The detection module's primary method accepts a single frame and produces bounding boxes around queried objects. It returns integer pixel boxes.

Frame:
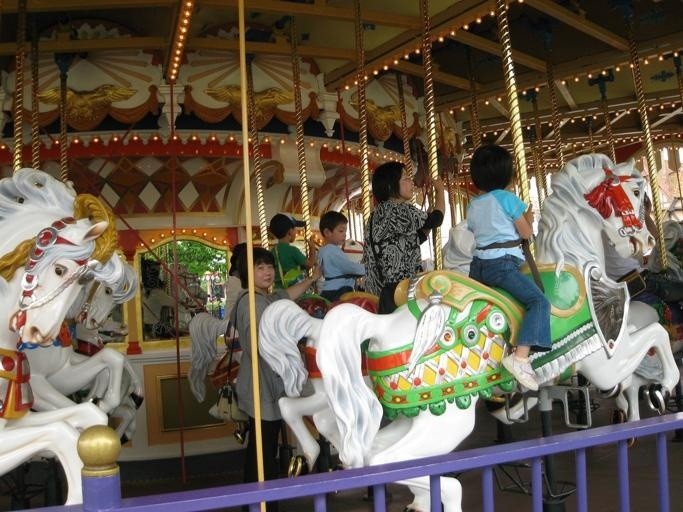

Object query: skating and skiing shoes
[503,352,539,392]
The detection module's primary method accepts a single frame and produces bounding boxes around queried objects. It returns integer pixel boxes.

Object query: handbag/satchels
[377,282,400,313]
[216,383,250,422]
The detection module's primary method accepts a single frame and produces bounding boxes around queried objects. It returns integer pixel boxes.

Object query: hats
[270,213,305,232]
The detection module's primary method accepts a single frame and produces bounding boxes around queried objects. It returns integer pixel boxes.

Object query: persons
[361,160,445,295]
[465,144,552,393]
[270,213,316,288]
[225,243,246,327]
[231,247,321,511]
[141,259,180,339]
[318,211,365,301]
